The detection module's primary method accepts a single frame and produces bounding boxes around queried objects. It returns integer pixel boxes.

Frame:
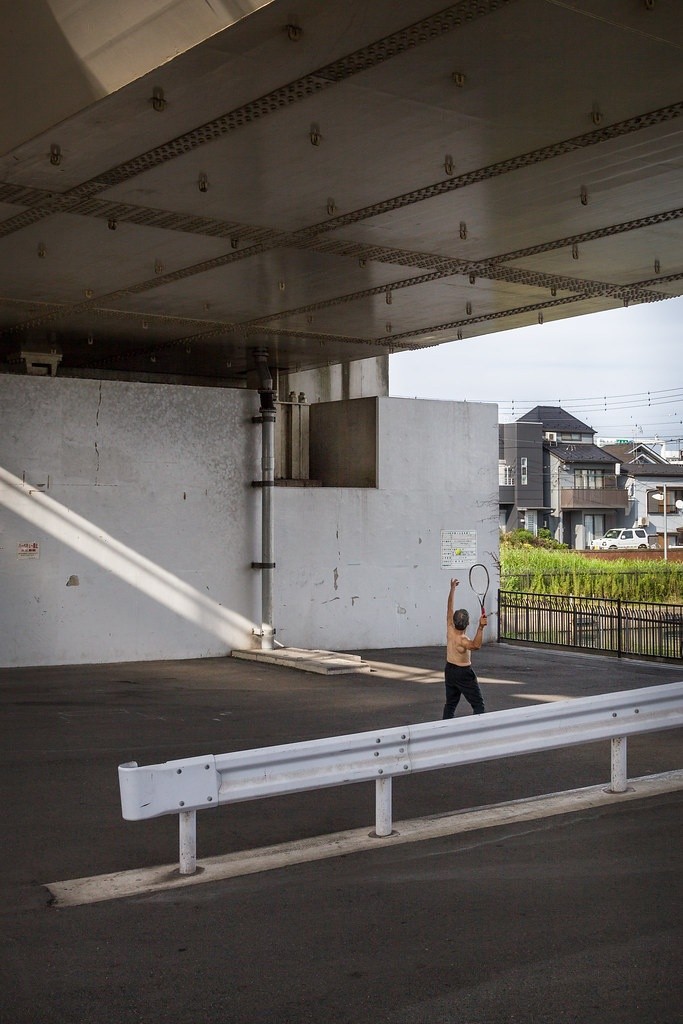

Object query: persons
[442,578,488,720]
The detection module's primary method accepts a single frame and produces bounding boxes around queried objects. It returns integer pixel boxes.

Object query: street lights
[652,492,667,559]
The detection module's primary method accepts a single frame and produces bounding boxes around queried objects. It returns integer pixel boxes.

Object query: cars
[591,528,650,550]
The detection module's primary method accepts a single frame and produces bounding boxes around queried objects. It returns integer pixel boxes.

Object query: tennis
[455,549,462,555]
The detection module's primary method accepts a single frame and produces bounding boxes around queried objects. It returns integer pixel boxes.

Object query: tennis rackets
[469,563,490,626]
